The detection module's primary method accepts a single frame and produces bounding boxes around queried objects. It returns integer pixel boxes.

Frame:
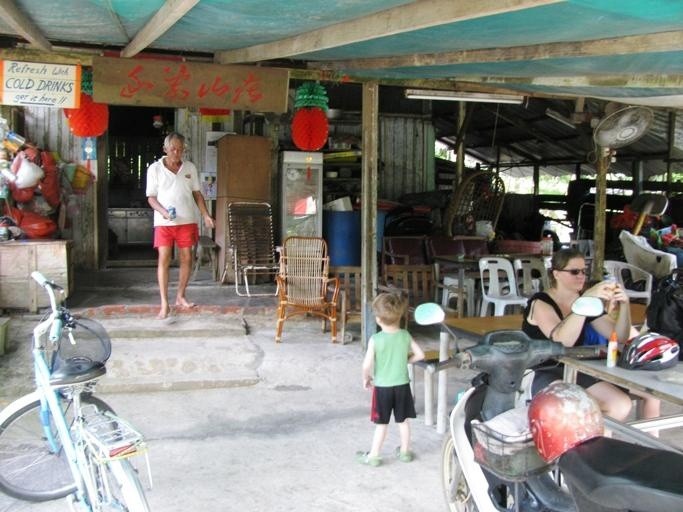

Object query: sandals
[394,445,411,463]
[354,450,380,466]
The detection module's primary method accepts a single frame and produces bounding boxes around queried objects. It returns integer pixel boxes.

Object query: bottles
[0,117,26,201]
[606,332,618,368]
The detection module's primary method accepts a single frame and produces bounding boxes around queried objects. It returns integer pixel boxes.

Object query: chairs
[619,229,677,282]
[274,237,341,340]
[222,200,279,297]
[514,257,548,297]
[603,260,651,304]
[479,257,528,316]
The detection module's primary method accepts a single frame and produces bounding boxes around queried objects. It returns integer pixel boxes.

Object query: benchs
[328,264,439,345]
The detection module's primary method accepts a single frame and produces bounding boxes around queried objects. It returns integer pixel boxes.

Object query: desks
[437,302,659,435]
[555,350,683,487]
[431,251,551,318]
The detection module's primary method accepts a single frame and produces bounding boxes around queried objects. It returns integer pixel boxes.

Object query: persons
[146,132,216,320]
[354,291,426,467]
[515,247,661,439]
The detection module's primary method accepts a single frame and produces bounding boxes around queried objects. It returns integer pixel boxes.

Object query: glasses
[559,267,586,276]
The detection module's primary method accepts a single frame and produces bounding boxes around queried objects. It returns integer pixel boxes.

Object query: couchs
[382,235,555,302]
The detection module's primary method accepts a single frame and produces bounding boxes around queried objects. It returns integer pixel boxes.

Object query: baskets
[46,315,112,401]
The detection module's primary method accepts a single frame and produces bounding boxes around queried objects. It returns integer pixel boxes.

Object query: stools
[413,350,458,425]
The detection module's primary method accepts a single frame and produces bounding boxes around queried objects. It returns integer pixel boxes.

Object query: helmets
[616,330,682,373]
[527,378,605,460]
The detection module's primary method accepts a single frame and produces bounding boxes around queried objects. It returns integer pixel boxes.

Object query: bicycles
[0,271,152,512]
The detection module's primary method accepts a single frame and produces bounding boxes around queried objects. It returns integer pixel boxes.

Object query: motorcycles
[415,297,681,512]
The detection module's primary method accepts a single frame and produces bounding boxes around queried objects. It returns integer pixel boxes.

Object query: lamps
[152,107,163,129]
[402,87,525,106]
[544,108,577,130]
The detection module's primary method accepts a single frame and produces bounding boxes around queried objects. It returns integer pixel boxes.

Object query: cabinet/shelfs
[215,133,274,282]
[108,207,157,246]
[1,238,74,314]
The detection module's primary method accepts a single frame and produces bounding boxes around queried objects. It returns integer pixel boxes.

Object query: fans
[592,103,656,150]
